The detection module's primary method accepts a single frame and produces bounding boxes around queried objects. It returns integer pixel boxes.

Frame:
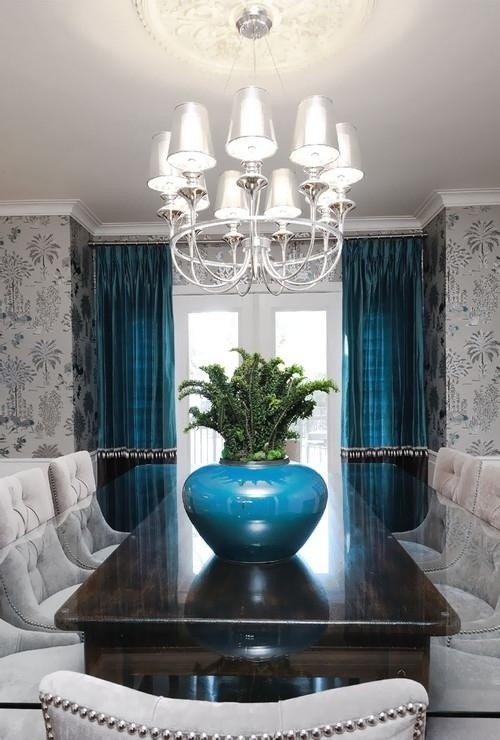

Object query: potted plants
[177,347,339,569]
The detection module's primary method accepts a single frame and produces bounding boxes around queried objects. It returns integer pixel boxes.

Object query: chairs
[38,669,429,739]
[0,449,132,739]
[389,448,500,739]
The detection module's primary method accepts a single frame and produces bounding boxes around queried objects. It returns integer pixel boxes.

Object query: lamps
[136,1,375,296]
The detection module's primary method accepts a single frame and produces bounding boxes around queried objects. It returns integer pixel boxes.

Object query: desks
[55,473,462,739]
